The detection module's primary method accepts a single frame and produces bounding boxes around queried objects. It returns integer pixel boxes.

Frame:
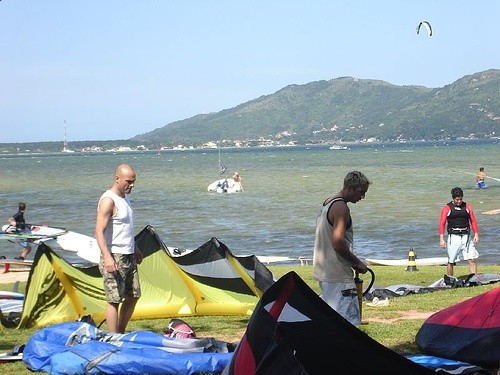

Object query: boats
[329,145,347,149]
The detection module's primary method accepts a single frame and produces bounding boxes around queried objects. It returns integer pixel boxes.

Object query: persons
[312,170,368,328]
[8,202,31,261]
[439,188,480,276]
[97,164,143,334]
[476,167,487,190]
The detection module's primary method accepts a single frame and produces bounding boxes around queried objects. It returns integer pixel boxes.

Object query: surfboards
[207,178,236,192]
[365,257,462,266]
[234,254,291,263]
[2,224,97,252]
[77,247,194,264]
